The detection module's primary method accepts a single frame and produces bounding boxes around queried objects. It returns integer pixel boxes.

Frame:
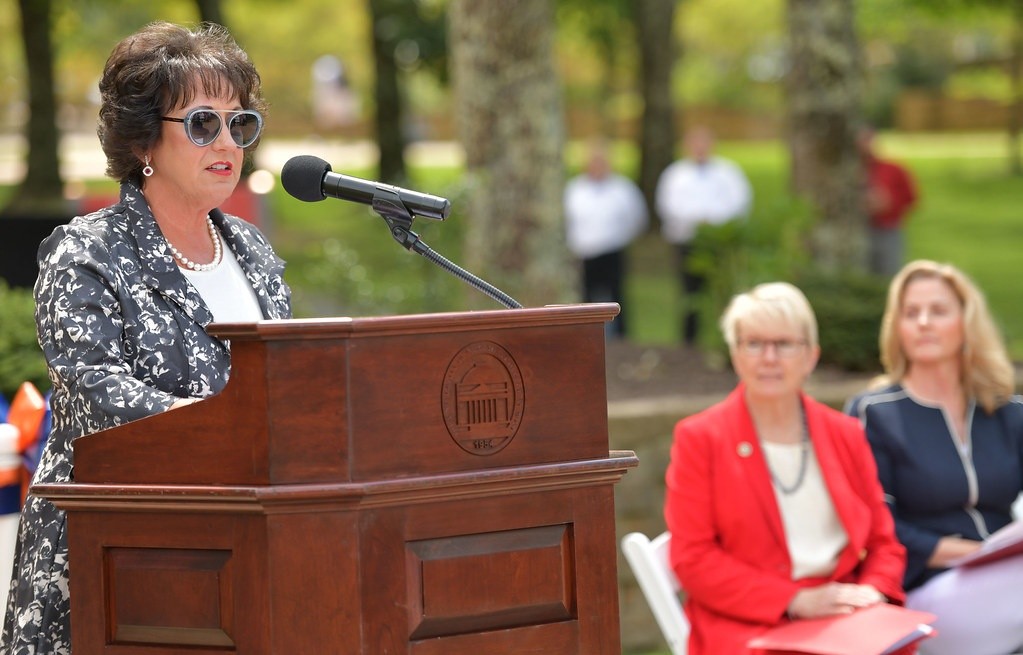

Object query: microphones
[281,155,451,222]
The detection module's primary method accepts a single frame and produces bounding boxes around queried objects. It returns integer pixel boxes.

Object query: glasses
[736,335,810,358]
[160,106,263,148]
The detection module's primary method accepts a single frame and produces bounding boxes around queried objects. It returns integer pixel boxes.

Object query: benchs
[621,526,694,654]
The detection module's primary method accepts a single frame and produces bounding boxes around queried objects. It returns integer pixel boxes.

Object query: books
[946,523,1023,567]
[747,599,936,655]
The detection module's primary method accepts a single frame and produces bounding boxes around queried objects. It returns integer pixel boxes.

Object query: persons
[565,126,923,359]
[664,283,919,655]
[0,22,292,655]
[844,257,1023,655]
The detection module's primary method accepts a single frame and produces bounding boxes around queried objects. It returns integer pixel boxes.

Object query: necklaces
[166,214,221,271]
[767,401,810,493]
